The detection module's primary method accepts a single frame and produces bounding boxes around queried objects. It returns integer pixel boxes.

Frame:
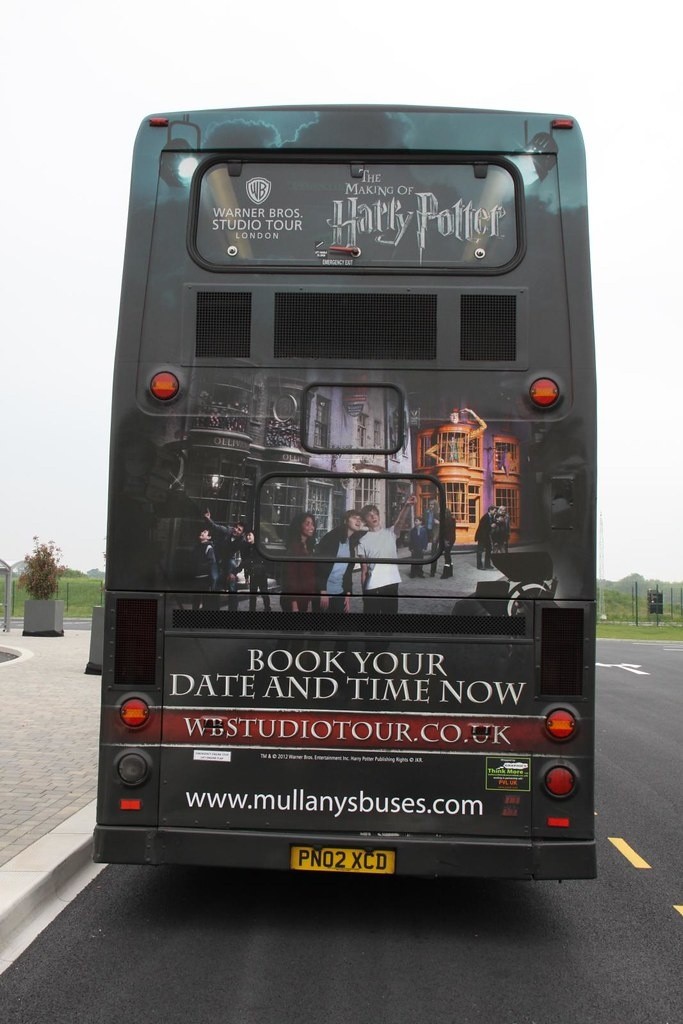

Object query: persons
[409,499,457,580]
[357,494,418,614]
[279,512,317,612]
[193,508,272,611]
[474,506,512,571]
[311,510,361,613]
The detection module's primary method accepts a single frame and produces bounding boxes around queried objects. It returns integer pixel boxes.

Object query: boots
[441,563,453,579]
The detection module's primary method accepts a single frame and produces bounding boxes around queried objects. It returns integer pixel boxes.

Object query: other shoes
[486,565,494,569]
[478,567,486,570]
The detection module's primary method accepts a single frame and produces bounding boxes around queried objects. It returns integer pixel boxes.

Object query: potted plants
[17,535,68,639]
[85,551,106,675]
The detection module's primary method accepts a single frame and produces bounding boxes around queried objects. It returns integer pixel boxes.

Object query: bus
[90,104,599,885]
